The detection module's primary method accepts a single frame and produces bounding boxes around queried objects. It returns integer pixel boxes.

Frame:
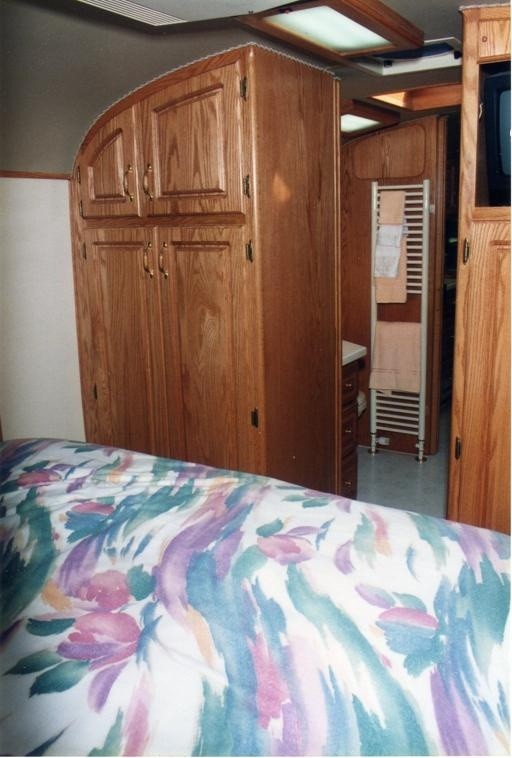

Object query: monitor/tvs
[483,69,511,207]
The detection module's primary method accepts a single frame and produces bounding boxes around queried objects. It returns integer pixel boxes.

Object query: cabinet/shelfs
[70,222,338,498]
[342,340,367,500]
[69,41,340,222]
[447,208,512,535]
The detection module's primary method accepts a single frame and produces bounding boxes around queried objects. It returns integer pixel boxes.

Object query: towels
[367,321,422,396]
[371,190,408,304]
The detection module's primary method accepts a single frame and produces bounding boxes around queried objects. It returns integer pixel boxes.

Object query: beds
[0,438,510,756]
